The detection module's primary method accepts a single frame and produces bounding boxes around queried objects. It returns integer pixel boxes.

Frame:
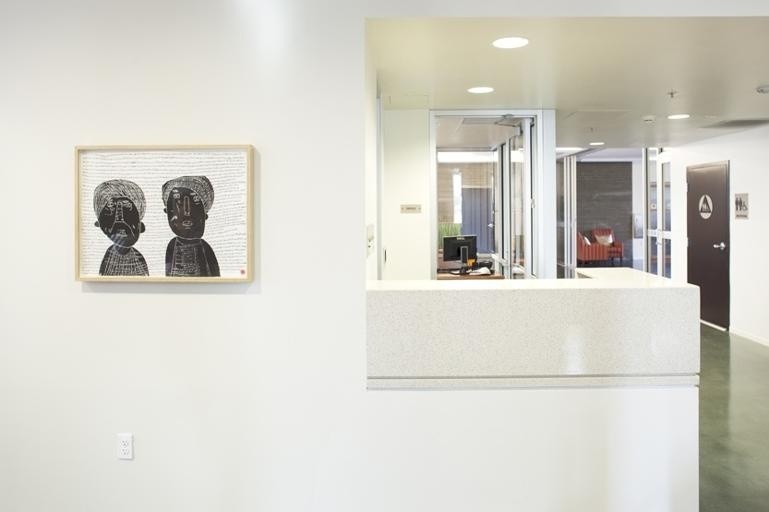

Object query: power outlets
[114,432,135,461]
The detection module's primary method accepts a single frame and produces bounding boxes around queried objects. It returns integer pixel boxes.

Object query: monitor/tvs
[442,234,478,275]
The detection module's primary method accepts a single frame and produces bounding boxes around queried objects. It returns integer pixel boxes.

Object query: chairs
[577,228,624,267]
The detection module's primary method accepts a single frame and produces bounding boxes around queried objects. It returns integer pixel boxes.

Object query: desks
[437,259,505,280]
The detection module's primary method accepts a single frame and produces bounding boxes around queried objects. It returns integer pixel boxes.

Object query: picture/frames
[73,145,256,284]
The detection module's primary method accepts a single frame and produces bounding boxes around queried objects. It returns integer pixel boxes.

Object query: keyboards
[437,268,460,273]
